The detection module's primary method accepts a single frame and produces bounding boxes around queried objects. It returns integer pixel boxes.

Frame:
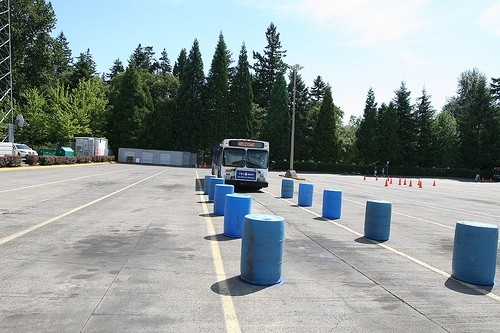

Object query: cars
[14,143,38,158]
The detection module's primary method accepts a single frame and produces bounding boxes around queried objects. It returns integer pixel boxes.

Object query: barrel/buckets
[322,189,342,220]
[223,192,252,238]
[213,184,234,216]
[204,175,217,195]
[451,220,498,286]
[298,183,313,207]
[208,178,225,201]
[281,178,294,199]
[364,200,392,241]
[240,213,285,285]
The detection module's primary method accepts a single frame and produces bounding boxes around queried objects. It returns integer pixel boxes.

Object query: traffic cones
[418,179,423,188]
[376,176,378,180]
[433,180,436,186]
[364,176,366,180]
[398,177,406,185]
[388,176,389,181]
[490,177,492,183]
[390,178,392,184]
[482,177,484,182]
[385,180,388,186]
[409,180,412,187]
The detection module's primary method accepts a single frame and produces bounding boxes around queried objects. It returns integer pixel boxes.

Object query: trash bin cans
[57,147,74,157]
[38,149,56,156]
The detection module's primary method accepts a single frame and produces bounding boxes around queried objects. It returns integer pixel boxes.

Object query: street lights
[284,63,305,176]
[204,162,206,168]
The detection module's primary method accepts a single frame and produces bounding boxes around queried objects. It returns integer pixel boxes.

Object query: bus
[212,138,270,191]
